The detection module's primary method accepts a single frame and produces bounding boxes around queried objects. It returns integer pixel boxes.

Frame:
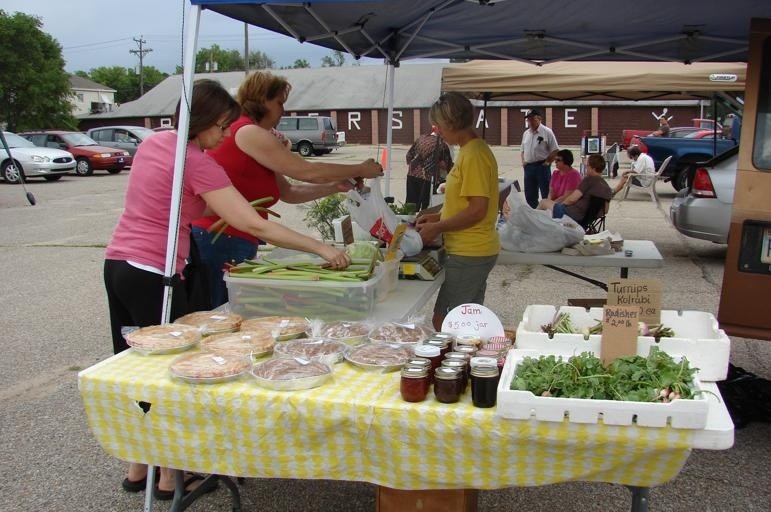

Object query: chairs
[577,195,606,234]
[620,155,673,201]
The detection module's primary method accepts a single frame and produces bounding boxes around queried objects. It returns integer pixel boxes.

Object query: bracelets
[652,132,657,137]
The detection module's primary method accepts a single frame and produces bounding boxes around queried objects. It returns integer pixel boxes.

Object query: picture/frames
[601,161,610,178]
[585,136,601,155]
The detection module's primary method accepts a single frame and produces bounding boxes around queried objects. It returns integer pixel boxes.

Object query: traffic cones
[379,148,391,171]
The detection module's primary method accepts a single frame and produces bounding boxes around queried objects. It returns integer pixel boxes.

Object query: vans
[274,116,339,156]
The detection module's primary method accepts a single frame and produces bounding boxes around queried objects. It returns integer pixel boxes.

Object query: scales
[398,251,444,281]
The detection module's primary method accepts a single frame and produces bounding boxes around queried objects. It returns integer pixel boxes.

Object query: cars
[15,130,133,176]
[0,130,77,185]
[668,132,771,246]
[87,125,156,167]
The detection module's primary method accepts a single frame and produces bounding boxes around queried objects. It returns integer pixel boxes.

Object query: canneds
[399,332,512,408]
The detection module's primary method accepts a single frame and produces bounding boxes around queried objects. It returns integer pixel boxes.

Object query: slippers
[154,473,217,500]
[122,469,160,491]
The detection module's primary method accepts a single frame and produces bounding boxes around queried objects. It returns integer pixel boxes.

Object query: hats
[523,109,541,118]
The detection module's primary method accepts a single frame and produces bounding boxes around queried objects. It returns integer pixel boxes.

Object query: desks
[495,239,664,295]
[76,347,734,512]
[432,178,520,215]
[211,271,446,323]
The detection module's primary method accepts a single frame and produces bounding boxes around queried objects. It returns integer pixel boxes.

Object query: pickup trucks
[334,130,345,147]
[621,116,741,191]
[622,119,726,153]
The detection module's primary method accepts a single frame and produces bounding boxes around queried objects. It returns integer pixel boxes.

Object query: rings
[336,262,340,265]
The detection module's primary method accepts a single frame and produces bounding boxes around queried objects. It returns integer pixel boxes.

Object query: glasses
[217,123,230,131]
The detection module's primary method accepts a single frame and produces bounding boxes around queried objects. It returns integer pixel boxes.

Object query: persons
[414,91,501,332]
[535,154,612,222]
[103,79,351,500]
[406,129,455,214]
[520,112,559,209]
[610,146,656,198]
[647,116,670,138]
[547,149,583,201]
[731,110,743,144]
[191,71,384,311]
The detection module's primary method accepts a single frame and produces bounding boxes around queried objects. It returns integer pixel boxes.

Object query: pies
[316,319,371,337]
[275,337,344,358]
[170,349,253,378]
[254,356,331,381]
[369,323,423,342]
[346,341,408,365]
[239,315,308,336]
[126,323,201,350]
[199,331,275,356]
[174,311,242,333]
